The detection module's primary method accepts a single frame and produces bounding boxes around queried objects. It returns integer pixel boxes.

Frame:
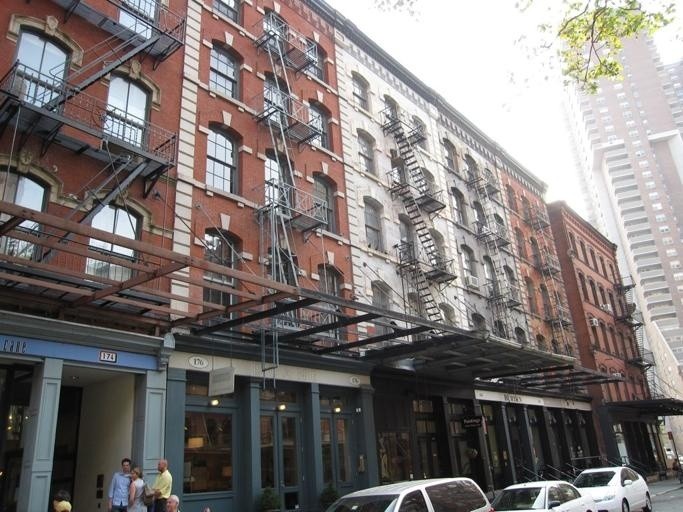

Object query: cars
[679,455,683,467]
[573,466,653,511]
[487,479,600,512]
[409,468,427,480]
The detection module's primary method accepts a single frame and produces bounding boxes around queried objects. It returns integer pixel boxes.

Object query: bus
[664,446,674,459]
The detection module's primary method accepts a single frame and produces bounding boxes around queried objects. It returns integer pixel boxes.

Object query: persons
[108,458,132,512]
[202,505,213,512]
[165,495,181,512]
[128,466,148,512]
[148,459,172,512]
[52,490,72,512]
[672,459,679,480]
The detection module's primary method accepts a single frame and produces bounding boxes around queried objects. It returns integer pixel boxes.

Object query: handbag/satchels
[140,478,154,508]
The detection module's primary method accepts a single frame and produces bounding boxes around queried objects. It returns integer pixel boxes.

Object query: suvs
[263,463,294,482]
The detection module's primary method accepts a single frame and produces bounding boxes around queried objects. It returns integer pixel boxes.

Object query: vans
[326,477,495,511]
